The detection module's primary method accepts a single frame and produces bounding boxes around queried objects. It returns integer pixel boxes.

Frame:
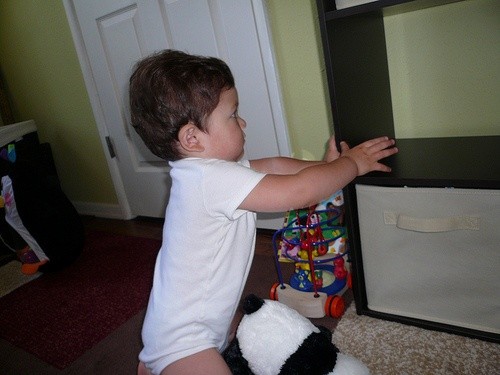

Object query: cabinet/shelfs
[311,0,500,345]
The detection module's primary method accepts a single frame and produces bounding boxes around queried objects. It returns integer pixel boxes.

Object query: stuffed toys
[221,294,369,375]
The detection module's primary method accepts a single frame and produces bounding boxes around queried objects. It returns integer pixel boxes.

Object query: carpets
[0,229,500,375]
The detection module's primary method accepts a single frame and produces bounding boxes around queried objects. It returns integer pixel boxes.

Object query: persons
[129,49,401,375]
